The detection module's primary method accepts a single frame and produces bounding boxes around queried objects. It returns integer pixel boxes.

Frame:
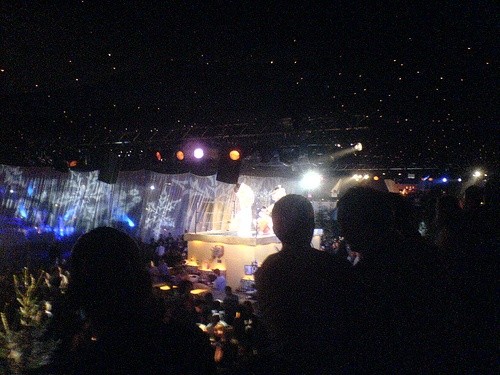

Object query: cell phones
[245,265,258,275]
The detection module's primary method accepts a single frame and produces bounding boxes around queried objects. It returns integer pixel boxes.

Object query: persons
[2,175,500,375]
